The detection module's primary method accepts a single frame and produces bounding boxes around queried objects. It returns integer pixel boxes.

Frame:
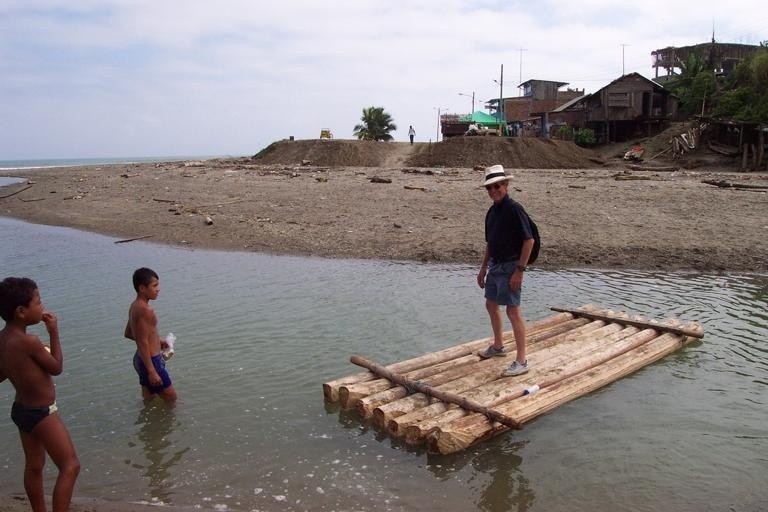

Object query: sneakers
[501,359,529,377]
[478,345,507,359]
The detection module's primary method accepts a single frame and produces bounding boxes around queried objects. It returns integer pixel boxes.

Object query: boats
[322,302,705,457]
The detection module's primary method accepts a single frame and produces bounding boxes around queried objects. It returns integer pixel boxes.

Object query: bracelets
[516,263,527,272]
[480,266,488,268]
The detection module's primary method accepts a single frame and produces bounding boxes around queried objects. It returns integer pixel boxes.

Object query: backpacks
[507,201,540,265]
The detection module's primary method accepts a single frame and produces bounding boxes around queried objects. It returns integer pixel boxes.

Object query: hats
[483,165,514,184]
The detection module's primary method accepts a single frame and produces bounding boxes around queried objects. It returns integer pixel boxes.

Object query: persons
[463,121,486,137]
[0,276,80,511]
[408,125,416,145]
[123,265,177,401]
[623,141,643,161]
[476,165,536,377]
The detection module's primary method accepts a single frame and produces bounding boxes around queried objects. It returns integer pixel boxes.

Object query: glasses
[486,185,505,189]
